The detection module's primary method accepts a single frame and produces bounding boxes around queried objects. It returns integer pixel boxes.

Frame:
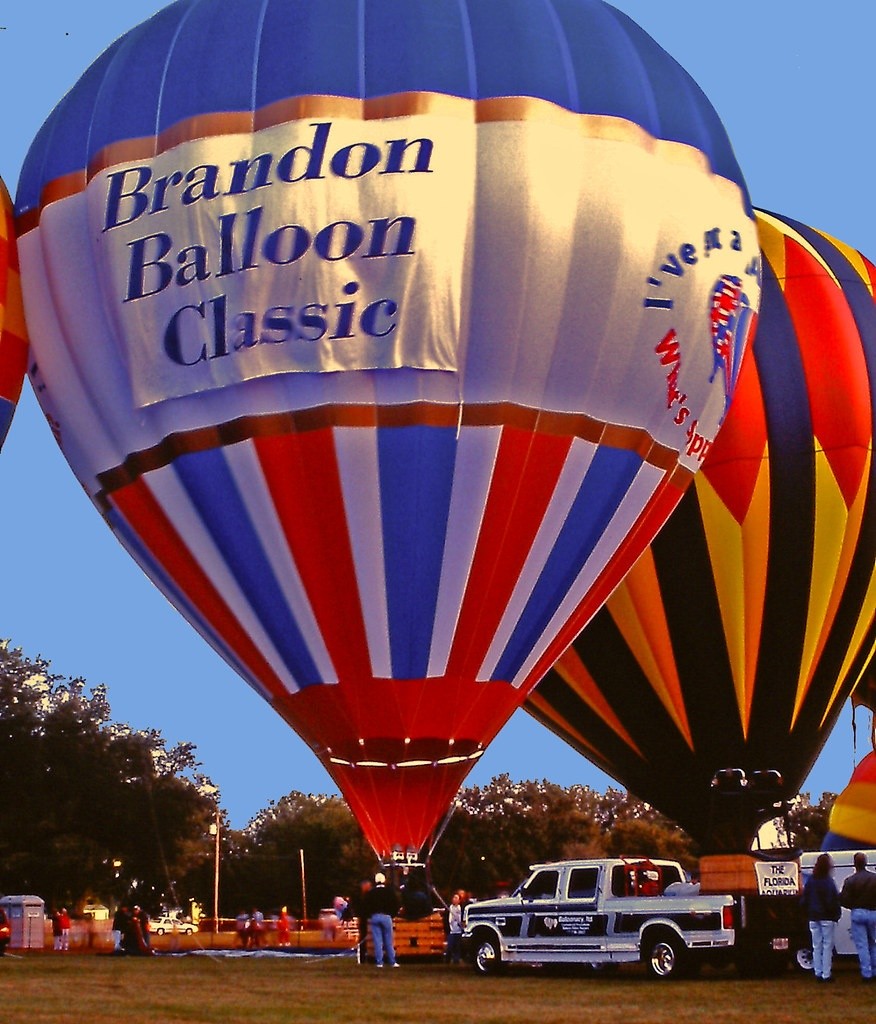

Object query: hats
[374,872,386,883]
[134,905,138,908]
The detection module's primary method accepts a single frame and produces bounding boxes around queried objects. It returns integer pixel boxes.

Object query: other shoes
[816,976,832,983]
[279,943,282,946]
[285,942,290,946]
[863,977,872,982]
[376,964,384,968]
[391,963,400,968]
[54,946,68,951]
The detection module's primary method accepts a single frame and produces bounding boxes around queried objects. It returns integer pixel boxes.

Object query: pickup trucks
[456,857,747,980]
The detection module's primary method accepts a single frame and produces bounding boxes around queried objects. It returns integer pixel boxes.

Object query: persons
[236,910,250,947]
[839,852,876,984]
[799,854,842,985]
[111,905,150,952]
[364,873,400,968]
[52,908,70,951]
[251,908,264,948]
[444,889,473,969]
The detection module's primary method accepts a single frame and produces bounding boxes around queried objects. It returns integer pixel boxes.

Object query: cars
[147,916,199,936]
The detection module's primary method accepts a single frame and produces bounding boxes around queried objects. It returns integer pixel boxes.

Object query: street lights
[209,808,220,934]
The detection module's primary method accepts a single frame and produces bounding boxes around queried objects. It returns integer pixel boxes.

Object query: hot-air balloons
[14,1,765,962]
[0,174,26,454]
[518,206,876,899]
